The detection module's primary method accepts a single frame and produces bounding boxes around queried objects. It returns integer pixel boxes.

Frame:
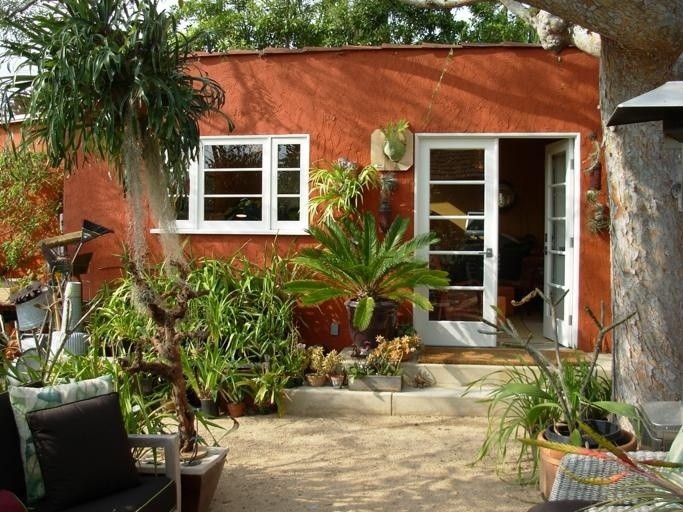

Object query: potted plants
[286,210,450,350]
[347,335,422,392]
[178,337,232,419]
[460,346,642,500]
[252,364,293,417]
[219,360,256,417]
[323,354,345,387]
[306,345,337,387]
[285,348,309,386]
[67,258,230,512]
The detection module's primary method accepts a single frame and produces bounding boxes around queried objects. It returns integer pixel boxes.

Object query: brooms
[55,218,115,297]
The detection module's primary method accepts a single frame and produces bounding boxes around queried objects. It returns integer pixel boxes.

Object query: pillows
[7,374,113,509]
[26,392,142,512]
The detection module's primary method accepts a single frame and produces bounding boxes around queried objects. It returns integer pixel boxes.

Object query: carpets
[417,344,593,365]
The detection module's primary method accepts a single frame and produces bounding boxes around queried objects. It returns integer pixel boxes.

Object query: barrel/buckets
[545,418,622,449]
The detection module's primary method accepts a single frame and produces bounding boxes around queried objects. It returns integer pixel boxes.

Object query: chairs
[0,380,182,512]
[524,412,683,512]
